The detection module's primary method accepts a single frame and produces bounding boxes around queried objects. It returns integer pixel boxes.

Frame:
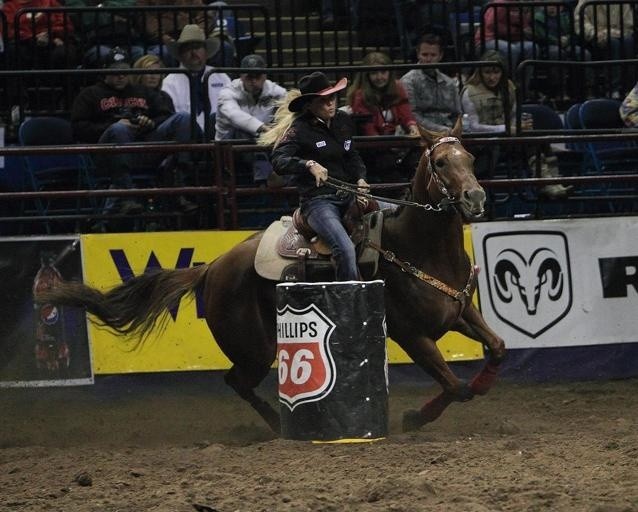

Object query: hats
[167,25,220,59]
[288,71,348,112]
[240,55,266,76]
[104,47,131,65]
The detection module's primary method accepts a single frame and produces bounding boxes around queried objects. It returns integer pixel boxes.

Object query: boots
[103,172,143,214]
[530,154,563,197]
[546,157,574,192]
[162,170,202,212]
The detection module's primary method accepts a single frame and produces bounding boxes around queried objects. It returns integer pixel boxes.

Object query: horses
[34,111,506,434]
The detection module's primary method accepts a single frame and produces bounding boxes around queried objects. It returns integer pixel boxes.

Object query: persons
[533,0,637,128]
[258,72,398,282]
[1,0,573,229]
